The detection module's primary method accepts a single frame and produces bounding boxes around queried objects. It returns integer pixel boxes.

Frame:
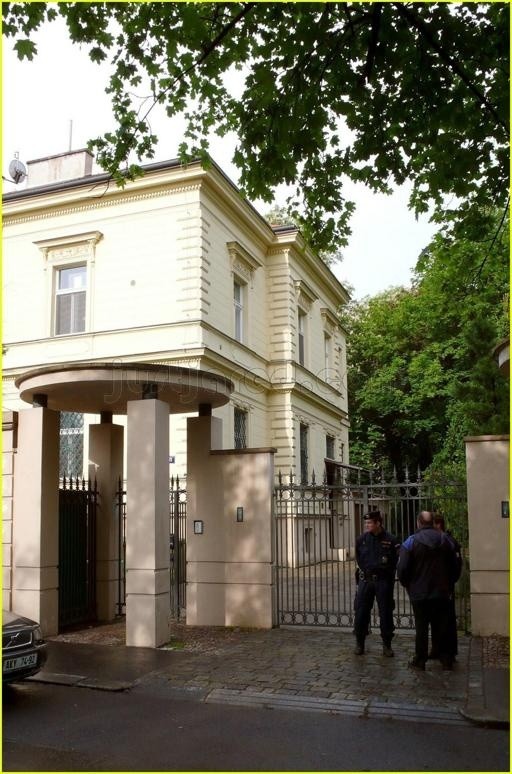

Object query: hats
[363,511,380,520]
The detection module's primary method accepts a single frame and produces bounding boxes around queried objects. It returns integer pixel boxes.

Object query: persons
[351,509,401,657]
[428,511,462,660]
[395,510,463,672]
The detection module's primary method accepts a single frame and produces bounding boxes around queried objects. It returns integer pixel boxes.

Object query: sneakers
[383,644,393,657]
[429,651,458,670]
[354,642,364,655]
[408,658,425,672]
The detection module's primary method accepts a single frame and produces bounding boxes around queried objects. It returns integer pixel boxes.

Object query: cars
[3,608,50,685]
